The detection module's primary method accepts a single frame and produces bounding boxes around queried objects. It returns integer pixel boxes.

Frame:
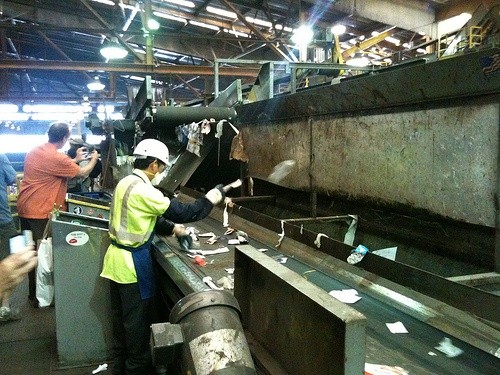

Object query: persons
[0,122,102,322]
[99,139,226,375]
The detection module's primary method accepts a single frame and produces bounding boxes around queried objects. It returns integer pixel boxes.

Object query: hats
[69,138,83,145]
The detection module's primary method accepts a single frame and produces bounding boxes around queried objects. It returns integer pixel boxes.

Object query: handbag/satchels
[36,217,54,309]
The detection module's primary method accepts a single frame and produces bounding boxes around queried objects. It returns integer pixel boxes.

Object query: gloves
[172,223,190,237]
[205,184,225,205]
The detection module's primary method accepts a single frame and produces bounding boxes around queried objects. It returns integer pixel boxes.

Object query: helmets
[133,139,171,167]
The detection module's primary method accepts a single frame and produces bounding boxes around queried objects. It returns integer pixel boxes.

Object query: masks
[144,170,164,185]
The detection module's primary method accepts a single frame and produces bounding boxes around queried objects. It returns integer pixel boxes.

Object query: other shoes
[28,297,54,307]
[146,366,157,375]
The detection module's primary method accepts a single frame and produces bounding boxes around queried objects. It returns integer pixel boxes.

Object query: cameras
[81,151,92,159]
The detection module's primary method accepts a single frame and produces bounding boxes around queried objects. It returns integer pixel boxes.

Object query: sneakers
[0,304,21,321]
